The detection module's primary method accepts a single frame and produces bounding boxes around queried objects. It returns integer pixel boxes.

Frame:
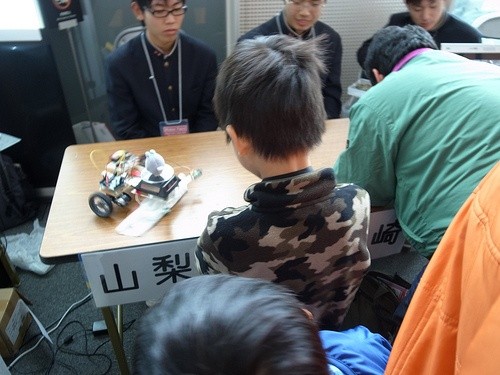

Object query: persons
[132,274,392,375]
[333,26,500,317]
[384,161,500,375]
[357,0,482,79]
[195,35,371,332]
[103,0,219,141]
[236,0,342,120]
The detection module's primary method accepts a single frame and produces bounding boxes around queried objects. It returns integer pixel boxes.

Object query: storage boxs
[0,287,32,360]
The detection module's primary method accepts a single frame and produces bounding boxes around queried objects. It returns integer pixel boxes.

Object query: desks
[39,115,404,375]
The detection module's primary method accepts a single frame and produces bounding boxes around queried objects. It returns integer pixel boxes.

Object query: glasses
[285,1,326,11]
[142,5,188,18]
